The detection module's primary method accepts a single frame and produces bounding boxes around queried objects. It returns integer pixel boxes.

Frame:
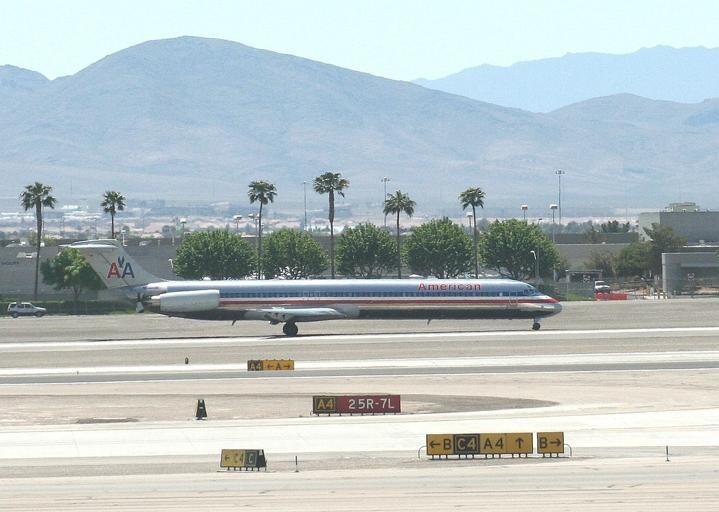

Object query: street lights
[121,229,128,247]
[379,175,392,229]
[233,214,243,233]
[554,168,566,223]
[248,213,262,237]
[466,211,474,232]
[521,203,529,220]
[179,217,188,231]
[548,203,560,280]
[302,180,308,228]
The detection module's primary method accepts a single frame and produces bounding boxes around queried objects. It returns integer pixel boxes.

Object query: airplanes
[55,237,567,334]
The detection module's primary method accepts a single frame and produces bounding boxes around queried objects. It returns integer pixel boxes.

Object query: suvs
[593,280,612,295]
[7,303,47,318]
